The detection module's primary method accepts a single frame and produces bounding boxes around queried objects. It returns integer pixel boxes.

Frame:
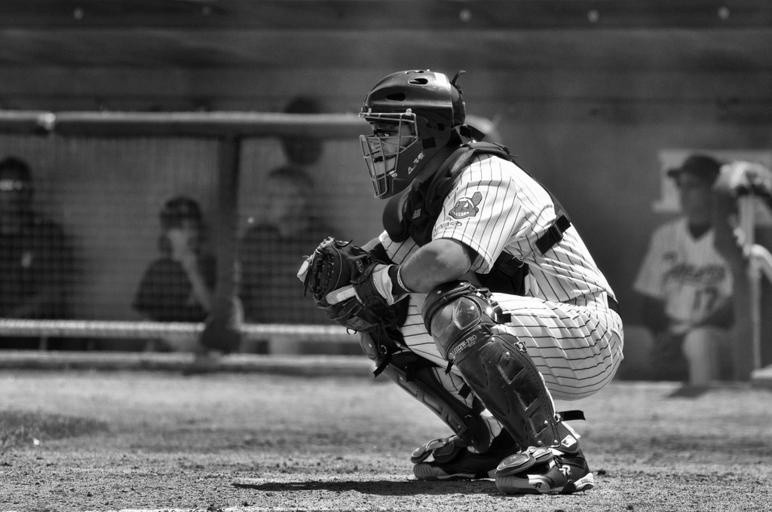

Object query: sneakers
[413,445,521,481]
[496,448,594,494]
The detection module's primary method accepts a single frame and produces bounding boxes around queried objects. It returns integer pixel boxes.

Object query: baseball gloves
[297,235,395,332]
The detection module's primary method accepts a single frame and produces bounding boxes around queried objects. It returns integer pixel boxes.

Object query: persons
[240,167,350,354]
[130,196,217,352]
[622,154,738,384]
[295,68,626,493]
[0,155,74,349]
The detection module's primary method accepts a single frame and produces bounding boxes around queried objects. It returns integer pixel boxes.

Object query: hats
[667,154,721,184]
[161,197,201,221]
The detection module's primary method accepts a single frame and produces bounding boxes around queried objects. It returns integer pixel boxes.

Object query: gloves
[325,263,414,308]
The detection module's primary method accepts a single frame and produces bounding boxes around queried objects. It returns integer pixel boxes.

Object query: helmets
[359,69,466,200]
